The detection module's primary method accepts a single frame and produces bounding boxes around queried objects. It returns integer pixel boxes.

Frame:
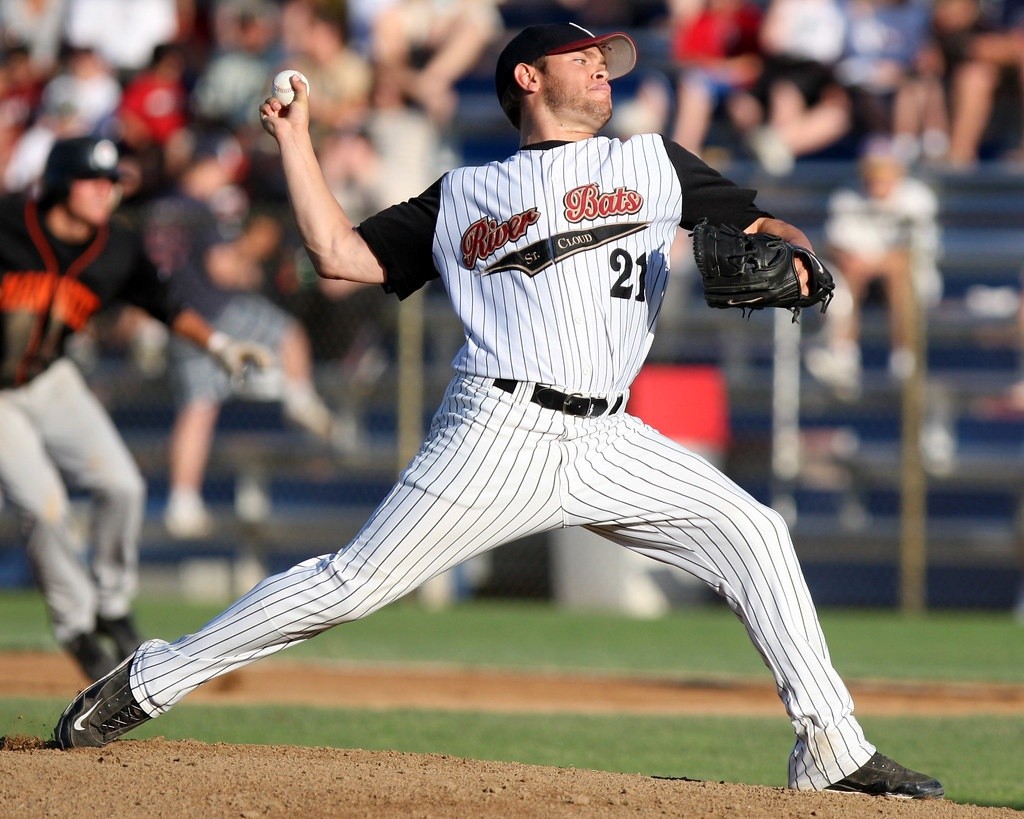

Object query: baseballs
[273,70,310,106]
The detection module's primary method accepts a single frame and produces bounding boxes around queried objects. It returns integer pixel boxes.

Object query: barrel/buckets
[545,351,730,612]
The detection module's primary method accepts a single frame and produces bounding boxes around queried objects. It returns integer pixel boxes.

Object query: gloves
[206,330,268,376]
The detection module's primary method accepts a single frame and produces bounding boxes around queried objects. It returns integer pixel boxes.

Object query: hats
[496,20,636,114]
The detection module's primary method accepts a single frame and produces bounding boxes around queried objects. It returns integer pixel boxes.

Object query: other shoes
[803,346,861,403]
[96,614,141,663]
[69,630,118,680]
[750,124,797,177]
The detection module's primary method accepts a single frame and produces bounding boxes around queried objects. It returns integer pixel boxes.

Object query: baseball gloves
[688,218,836,310]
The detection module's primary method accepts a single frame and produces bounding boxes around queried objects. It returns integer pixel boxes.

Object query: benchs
[0,155,1023,608]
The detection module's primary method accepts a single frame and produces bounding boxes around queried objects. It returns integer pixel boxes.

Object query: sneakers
[53,645,152,751]
[823,751,944,800]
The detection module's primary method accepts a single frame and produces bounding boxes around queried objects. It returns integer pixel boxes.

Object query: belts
[493,377,624,419]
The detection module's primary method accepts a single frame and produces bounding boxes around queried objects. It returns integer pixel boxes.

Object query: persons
[54,20,943,797]
[0,0,1024,539]
[0,136,271,683]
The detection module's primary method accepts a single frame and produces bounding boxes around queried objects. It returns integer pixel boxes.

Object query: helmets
[42,137,121,191]
